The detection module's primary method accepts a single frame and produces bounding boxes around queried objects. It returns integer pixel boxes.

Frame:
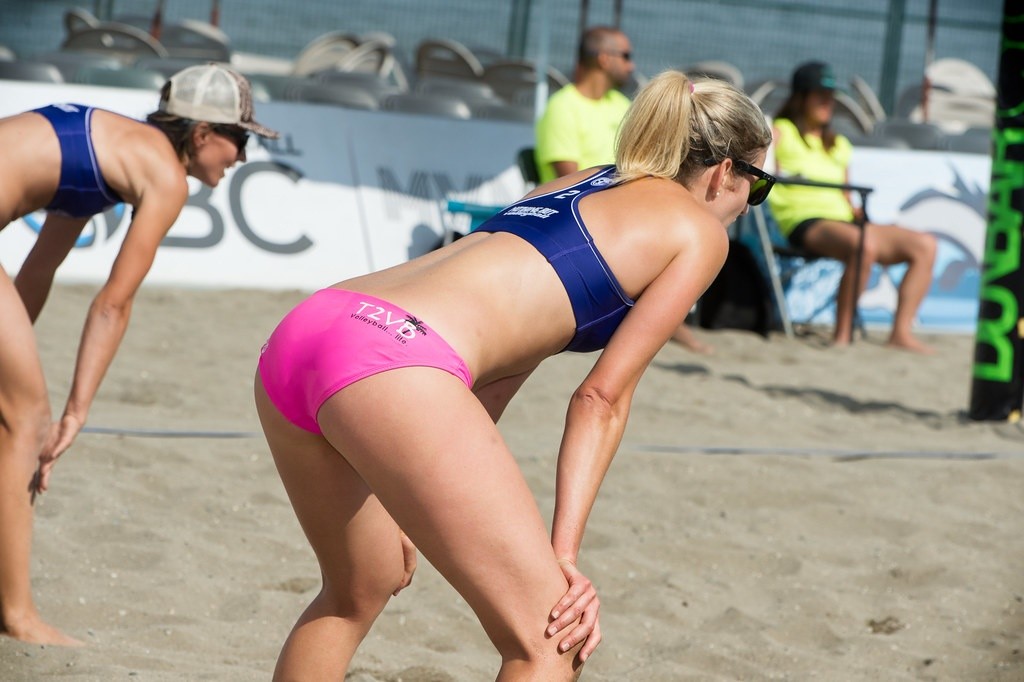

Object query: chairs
[746,185,875,340]
[0,8,998,152]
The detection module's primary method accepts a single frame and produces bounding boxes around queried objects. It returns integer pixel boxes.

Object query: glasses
[703,158,777,206]
[610,51,629,61]
[210,125,249,152]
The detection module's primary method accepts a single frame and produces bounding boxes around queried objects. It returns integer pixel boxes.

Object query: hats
[792,62,839,91]
[158,63,281,139]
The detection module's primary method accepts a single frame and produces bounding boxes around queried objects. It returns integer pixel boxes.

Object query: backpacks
[696,238,772,338]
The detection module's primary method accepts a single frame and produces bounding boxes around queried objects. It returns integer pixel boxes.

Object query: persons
[0,62,281,646]
[767,60,935,357]
[255,69,774,681]
[531,26,711,357]
